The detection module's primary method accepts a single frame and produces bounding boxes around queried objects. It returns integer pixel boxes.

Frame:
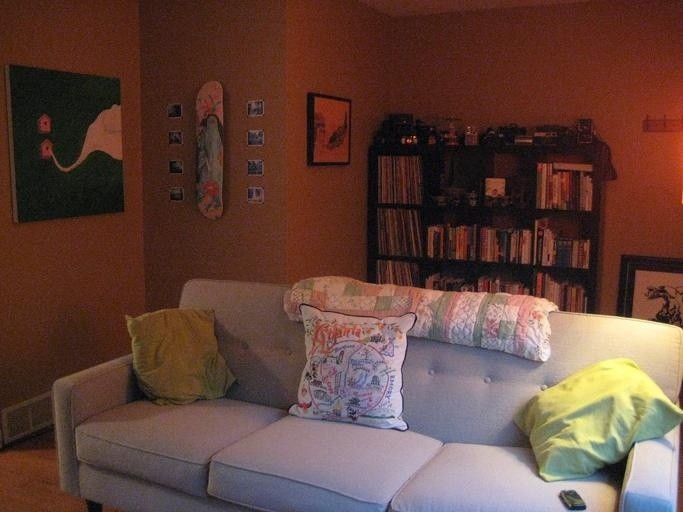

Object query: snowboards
[195,81,223,221]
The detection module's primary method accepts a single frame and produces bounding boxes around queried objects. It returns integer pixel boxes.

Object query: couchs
[55,280,683,511]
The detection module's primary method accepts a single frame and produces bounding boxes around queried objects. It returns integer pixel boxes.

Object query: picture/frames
[618,254,682,328]
[167,102,184,201]
[246,100,264,205]
[306,92,350,165]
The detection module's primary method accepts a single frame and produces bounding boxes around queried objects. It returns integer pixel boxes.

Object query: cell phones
[560,490,585,509]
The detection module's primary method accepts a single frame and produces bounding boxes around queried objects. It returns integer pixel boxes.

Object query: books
[377,156,593,315]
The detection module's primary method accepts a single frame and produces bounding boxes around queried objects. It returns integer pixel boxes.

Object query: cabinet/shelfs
[366,143,481,294]
[482,144,601,317]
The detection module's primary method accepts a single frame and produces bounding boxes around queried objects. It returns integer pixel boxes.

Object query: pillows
[513,356,682,483]
[288,302,416,431]
[122,306,236,407]
